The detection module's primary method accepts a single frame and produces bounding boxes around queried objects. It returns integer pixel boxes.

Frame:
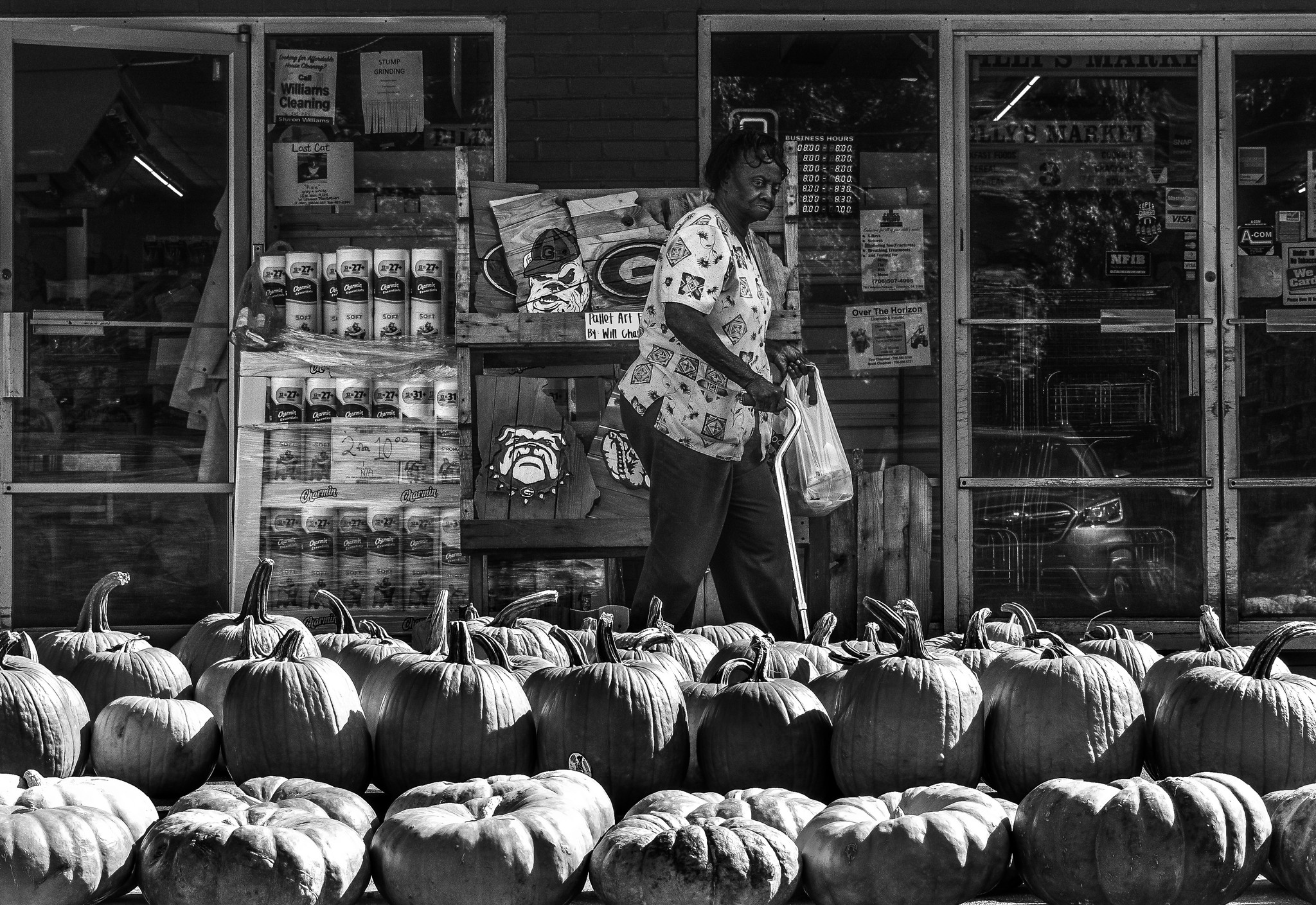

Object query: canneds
[254,247,462,613]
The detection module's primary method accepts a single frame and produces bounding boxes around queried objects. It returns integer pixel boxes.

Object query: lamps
[132,148,191,199]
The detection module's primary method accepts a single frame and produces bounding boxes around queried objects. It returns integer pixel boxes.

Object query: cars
[971,425,1140,618]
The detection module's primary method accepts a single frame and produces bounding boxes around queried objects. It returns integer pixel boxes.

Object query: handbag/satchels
[766,364,854,518]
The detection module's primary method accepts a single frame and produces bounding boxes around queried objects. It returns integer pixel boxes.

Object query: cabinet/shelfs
[233,354,466,638]
[449,142,810,635]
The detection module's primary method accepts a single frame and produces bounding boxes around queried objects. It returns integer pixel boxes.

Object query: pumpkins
[0,559,1316,905]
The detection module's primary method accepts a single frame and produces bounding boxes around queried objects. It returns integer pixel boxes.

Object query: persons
[619,125,814,643]
